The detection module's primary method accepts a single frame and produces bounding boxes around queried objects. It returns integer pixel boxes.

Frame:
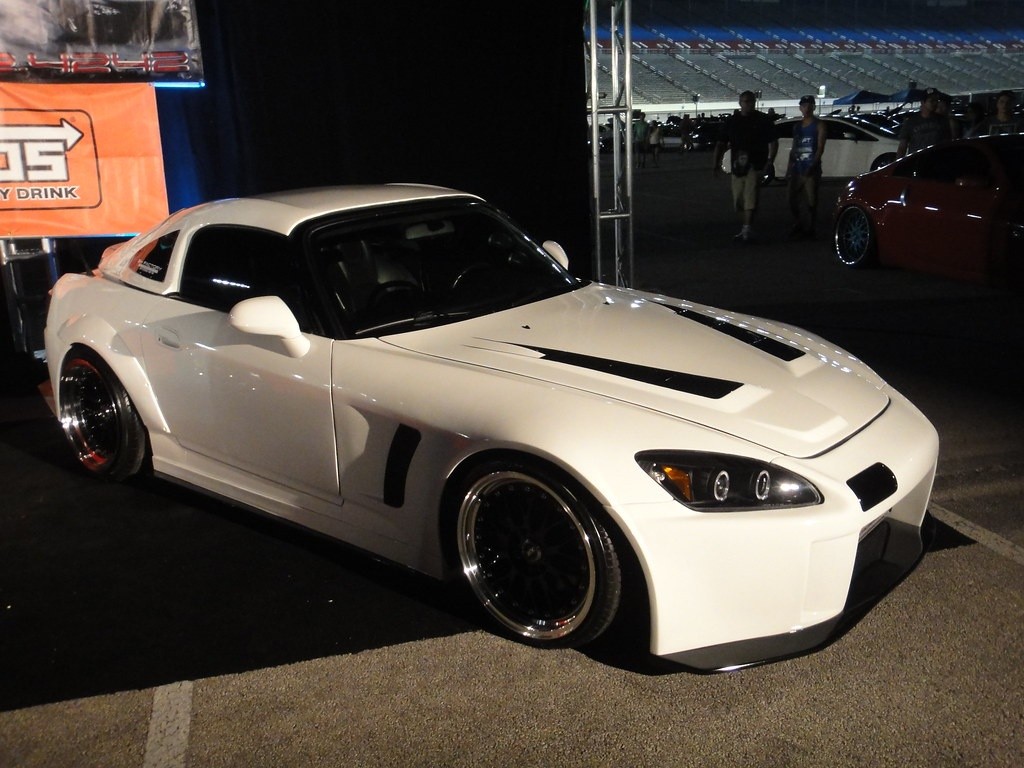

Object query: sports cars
[41,182,942,676]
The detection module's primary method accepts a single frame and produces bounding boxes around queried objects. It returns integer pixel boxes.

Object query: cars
[586,111,902,156]
[718,116,910,187]
[824,132,1024,285]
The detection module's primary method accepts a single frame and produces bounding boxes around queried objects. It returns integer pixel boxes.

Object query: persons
[712,90,778,243]
[895,88,1024,158]
[787,95,826,235]
[634,112,705,169]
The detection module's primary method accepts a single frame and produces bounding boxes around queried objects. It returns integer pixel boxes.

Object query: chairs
[333,240,417,333]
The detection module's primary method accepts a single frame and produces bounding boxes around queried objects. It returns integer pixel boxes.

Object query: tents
[832,89,950,115]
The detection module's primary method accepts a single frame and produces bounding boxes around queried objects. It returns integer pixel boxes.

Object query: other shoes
[810,228,817,240]
[787,224,802,237]
[734,225,752,240]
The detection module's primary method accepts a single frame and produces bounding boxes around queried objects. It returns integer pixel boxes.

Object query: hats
[921,88,939,99]
[799,95,815,105]
